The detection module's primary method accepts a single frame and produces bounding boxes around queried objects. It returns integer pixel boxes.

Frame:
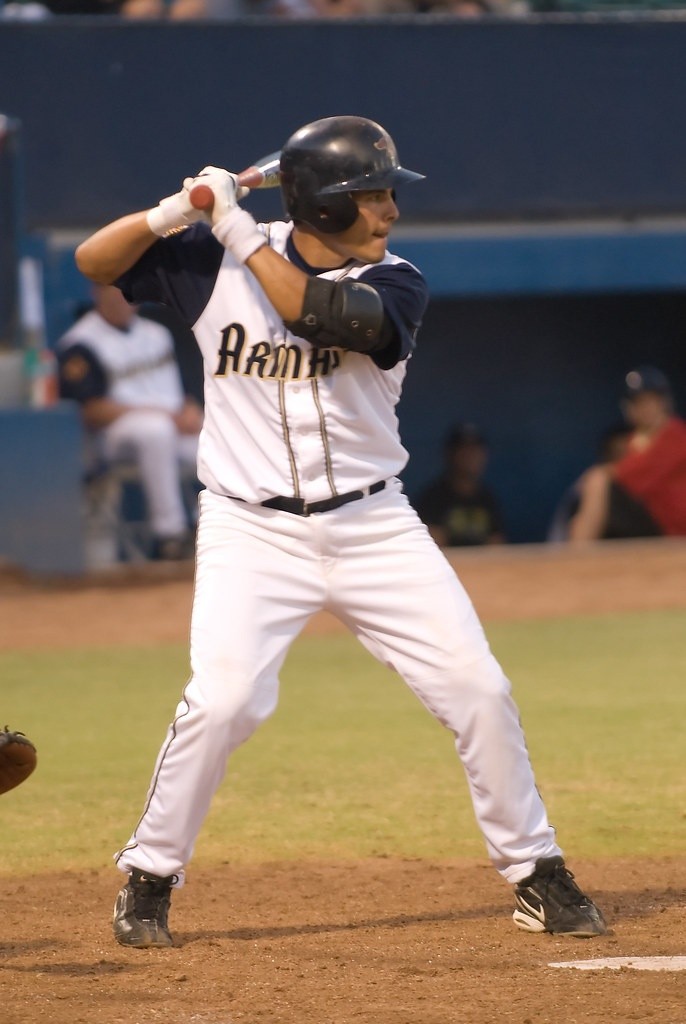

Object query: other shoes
[157,541,183,559]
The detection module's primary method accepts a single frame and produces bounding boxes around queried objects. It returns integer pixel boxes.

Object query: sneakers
[512,855,608,938]
[113,867,179,949]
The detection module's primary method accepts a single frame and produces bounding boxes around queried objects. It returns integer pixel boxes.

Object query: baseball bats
[189,146,284,211]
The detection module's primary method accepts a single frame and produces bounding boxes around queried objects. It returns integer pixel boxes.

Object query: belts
[227,480,385,517]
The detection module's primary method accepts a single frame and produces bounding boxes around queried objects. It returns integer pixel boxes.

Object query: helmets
[620,364,670,399]
[279,115,427,233]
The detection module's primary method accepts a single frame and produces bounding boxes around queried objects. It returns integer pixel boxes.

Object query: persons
[75,116,605,946]
[412,422,507,547]
[569,367,685,541]
[48,284,204,563]
[0,0,686,19]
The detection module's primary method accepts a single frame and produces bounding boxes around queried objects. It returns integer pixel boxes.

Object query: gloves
[183,166,268,266]
[146,166,250,239]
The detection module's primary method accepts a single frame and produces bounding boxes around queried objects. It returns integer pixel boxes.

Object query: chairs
[78,427,195,585]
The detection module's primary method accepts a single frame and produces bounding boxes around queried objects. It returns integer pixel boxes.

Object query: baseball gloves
[0,722,38,798]
